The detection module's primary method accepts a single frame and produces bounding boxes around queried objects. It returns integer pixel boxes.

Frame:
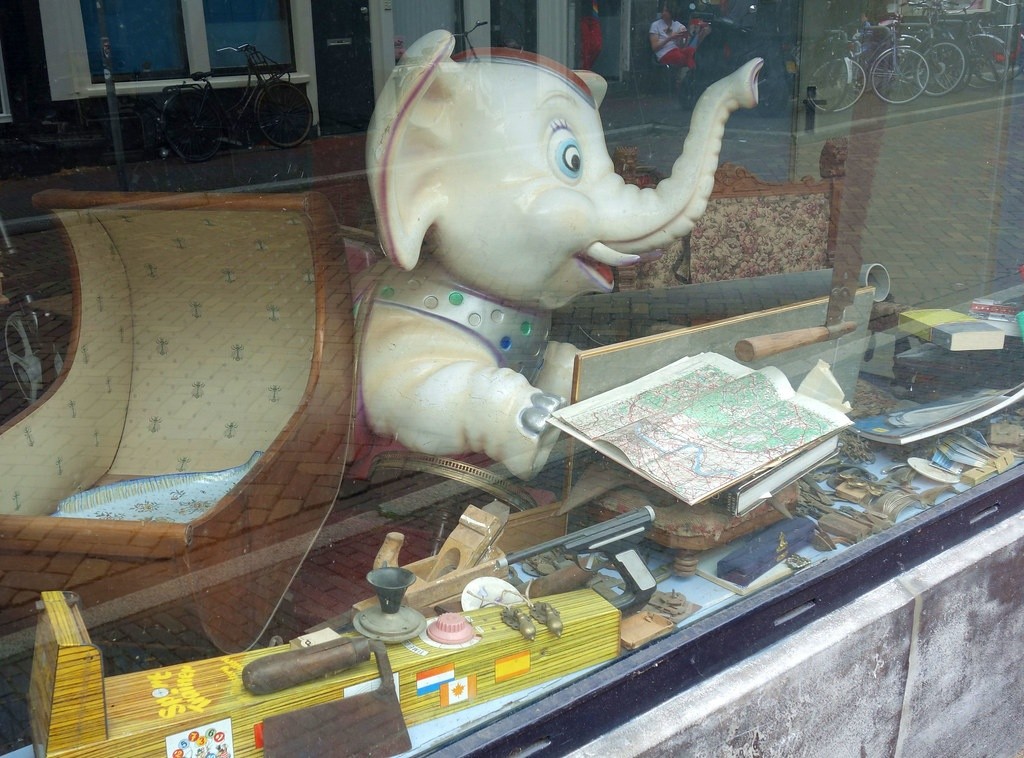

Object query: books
[540,290,1024,517]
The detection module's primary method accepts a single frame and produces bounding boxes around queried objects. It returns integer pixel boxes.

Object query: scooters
[678,0,797,117]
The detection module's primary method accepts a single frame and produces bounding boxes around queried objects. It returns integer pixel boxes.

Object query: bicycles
[397,19,489,129]
[158,42,314,163]
[804,0,1024,109]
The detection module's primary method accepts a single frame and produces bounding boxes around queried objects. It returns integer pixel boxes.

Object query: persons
[580,0,696,80]
